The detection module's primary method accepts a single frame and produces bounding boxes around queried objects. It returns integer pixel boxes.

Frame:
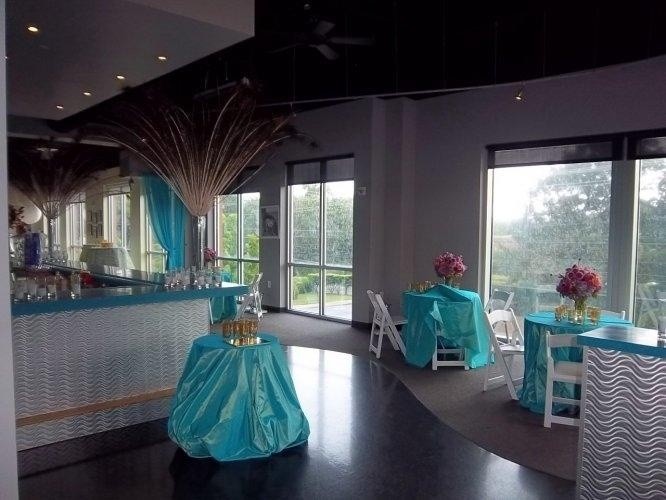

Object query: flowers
[432,251,469,278]
[555,264,601,300]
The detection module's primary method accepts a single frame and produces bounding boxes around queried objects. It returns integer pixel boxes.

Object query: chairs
[483,307,526,402]
[367,288,514,372]
[524,309,631,415]
[542,329,584,428]
[236,273,263,322]
[636,284,666,330]
[585,305,626,320]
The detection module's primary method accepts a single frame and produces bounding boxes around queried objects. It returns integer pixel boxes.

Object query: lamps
[512,84,528,106]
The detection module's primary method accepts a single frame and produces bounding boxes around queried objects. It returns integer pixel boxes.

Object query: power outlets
[268,281,271,288]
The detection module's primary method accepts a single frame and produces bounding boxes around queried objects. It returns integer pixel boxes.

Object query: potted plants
[8,163,95,261]
[94,81,302,273]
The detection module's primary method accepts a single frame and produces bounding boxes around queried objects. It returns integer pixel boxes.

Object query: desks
[168,332,310,463]
[207,271,237,325]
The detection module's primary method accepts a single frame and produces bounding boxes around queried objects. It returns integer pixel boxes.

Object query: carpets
[210,310,580,483]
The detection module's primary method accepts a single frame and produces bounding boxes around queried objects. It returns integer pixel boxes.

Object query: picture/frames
[259,204,279,239]
[85,210,102,237]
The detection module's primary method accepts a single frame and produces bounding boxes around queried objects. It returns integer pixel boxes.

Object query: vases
[444,275,460,291]
[574,296,587,316]
